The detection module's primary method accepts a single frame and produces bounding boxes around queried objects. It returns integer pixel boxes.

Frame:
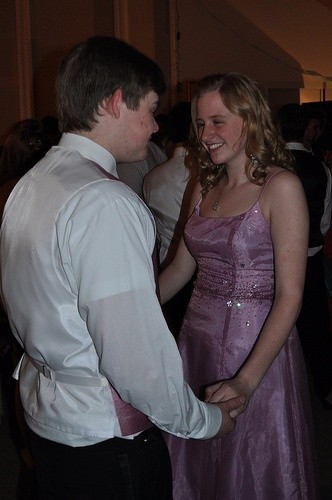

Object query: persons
[117,101,197,341]
[145,68,309,500]
[0,39,235,500]
[1,115,61,232]
[271,103,332,412]
